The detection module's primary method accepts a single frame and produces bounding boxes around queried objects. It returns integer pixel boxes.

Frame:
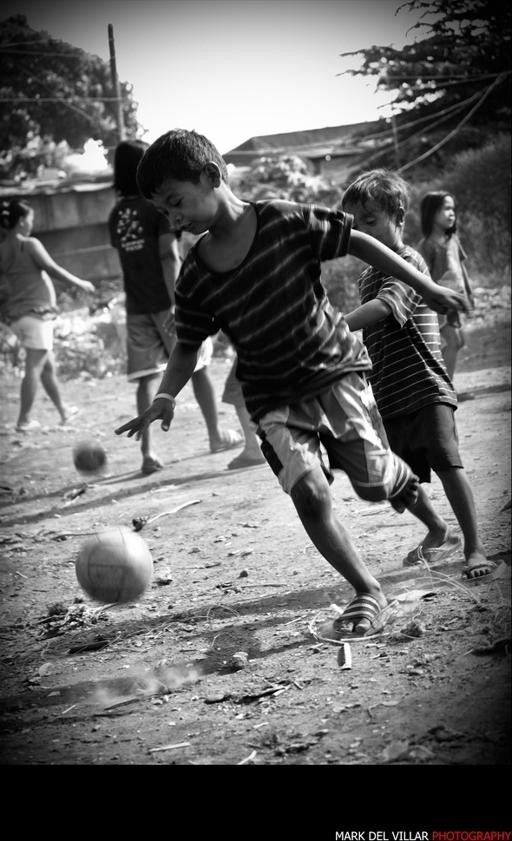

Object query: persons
[340,166,506,585]
[106,139,244,476]
[0,201,96,432]
[114,131,472,638]
[221,354,266,473]
[416,190,475,404]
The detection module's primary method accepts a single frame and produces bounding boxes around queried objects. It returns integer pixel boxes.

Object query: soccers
[75,443,104,473]
[75,527,155,606]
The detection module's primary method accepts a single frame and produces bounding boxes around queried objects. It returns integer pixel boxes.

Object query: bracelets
[152,393,176,410]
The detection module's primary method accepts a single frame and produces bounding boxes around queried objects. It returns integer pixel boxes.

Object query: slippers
[212,429,243,452]
[403,535,461,566]
[461,560,507,584]
[142,457,162,474]
[224,455,266,471]
[333,594,402,637]
[389,453,412,499]
